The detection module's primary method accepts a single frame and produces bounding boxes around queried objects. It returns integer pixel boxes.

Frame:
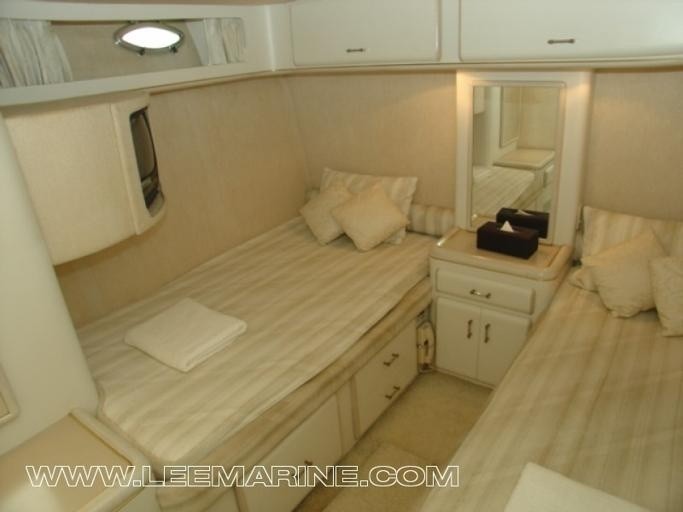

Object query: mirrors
[500,87,521,146]
[457,73,591,247]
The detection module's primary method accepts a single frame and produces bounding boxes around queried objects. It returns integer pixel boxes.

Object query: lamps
[115,21,185,56]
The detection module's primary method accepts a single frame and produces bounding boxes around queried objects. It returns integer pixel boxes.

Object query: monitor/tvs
[114,105,160,210]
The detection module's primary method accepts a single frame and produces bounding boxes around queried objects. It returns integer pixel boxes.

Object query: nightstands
[425,226,573,390]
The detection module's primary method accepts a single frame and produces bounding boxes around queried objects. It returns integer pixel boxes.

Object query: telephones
[417,320,435,365]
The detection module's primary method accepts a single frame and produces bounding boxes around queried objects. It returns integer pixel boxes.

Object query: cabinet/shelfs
[234,394,341,510]
[353,321,418,437]
[290,3,443,72]
[456,3,683,74]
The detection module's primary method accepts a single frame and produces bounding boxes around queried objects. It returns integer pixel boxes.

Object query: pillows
[569,203,681,343]
[297,166,451,254]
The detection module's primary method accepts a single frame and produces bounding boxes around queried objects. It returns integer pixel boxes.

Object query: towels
[121,295,248,374]
[504,458,652,512]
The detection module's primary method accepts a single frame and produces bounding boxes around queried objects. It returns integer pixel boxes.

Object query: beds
[420,266,682,510]
[69,208,443,509]
[473,164,542,219]
